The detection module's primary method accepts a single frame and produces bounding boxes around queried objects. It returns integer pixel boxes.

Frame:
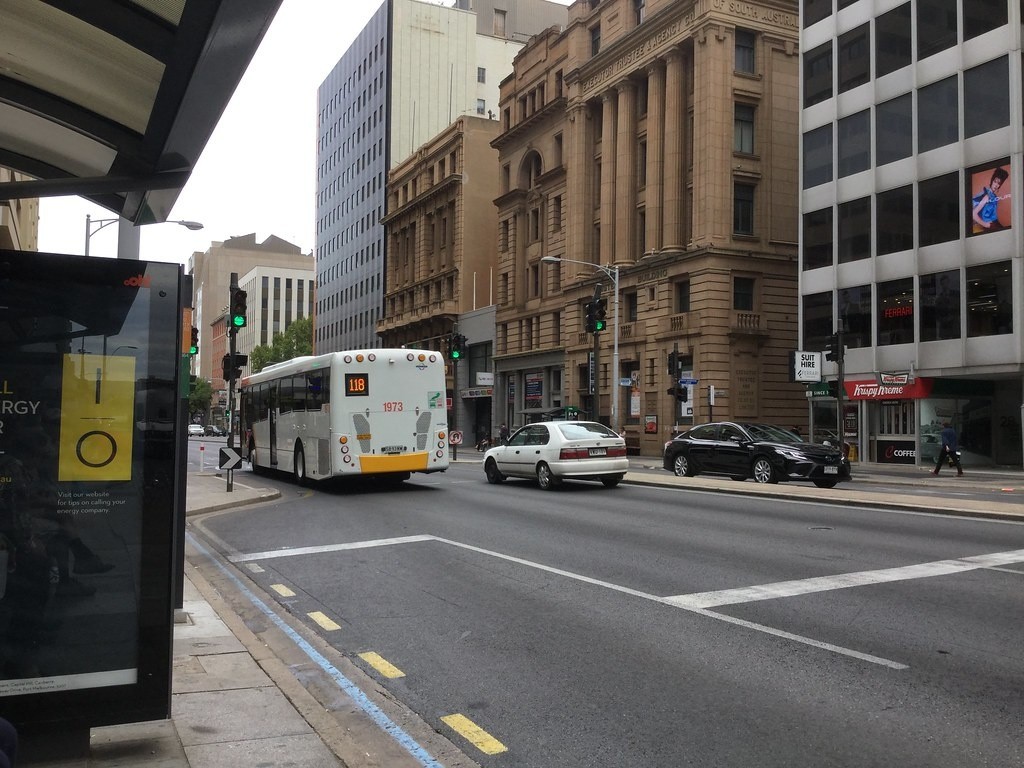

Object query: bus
[238,348,449,486]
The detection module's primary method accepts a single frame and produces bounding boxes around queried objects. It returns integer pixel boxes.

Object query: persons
[0,402,128,604]
[797,426,802,434]
[619,426,626,442]
[929,422,964,477]
[607,425,614,431]
[789,424,802,440]
[474,435,489,449]
[498,423,508,446]
[972,166,1010,232]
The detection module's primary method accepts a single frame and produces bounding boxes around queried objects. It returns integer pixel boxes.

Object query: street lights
[541,255,619,435]
[86,214,203,257]
[208,376,230,411]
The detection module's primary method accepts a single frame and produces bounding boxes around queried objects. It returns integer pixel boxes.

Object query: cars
[188,425,205,438]
[662,419,849,491]
[484,419,628,490]
[205,425,226,437]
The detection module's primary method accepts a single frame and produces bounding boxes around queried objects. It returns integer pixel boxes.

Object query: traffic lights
[450,333,462,361]
[232,288,247,327]
[445,335,451,358]
[225,410,229,417]
[190,328,199,355]
[594,299,606,332]
[825,331,841,363]
[585,305,592,334]
